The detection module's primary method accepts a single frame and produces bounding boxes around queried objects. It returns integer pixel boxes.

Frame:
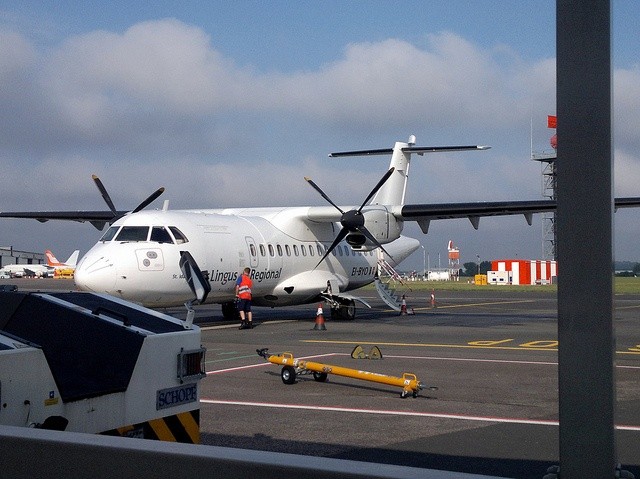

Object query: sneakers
[245,324,253,328]
[239,325,247,329]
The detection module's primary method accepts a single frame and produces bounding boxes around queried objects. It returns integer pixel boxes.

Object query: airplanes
[0,135,640,323]
[0,249,80,279]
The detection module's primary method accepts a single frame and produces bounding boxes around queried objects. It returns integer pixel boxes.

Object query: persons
[235,268,253,330]
